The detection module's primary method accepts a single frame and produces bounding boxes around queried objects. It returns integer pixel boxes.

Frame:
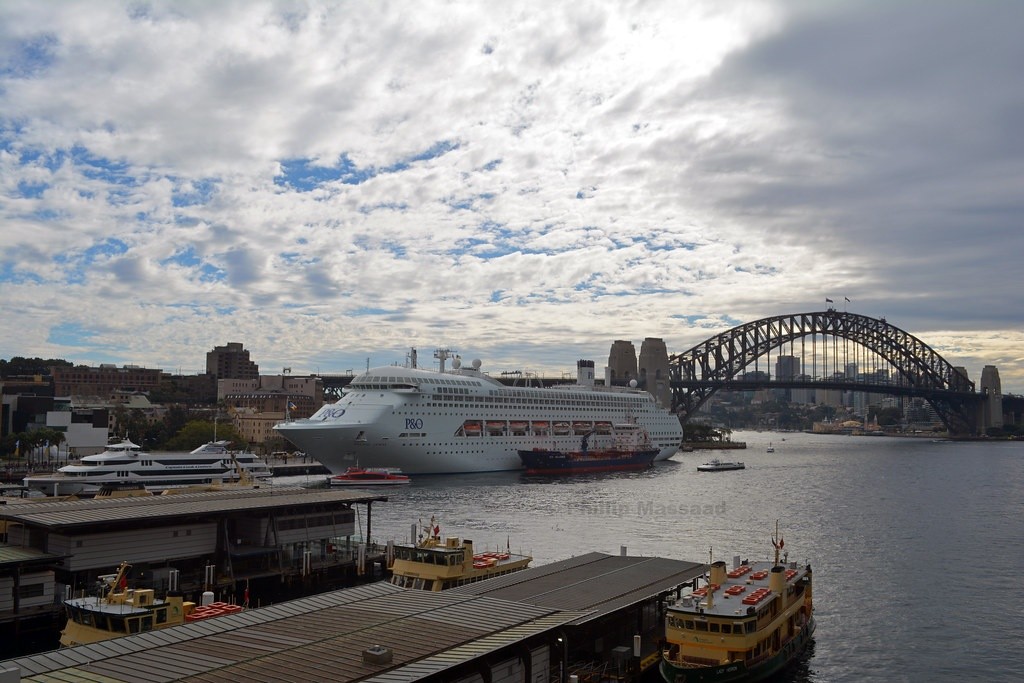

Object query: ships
[270,344,685,474]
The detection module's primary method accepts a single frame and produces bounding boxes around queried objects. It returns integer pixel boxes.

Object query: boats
[486,421,506,432]
[518,423,662,477]
[658,518,819,683]
[386,514,534,591]
[510,422,528,434]
[55,560,244,650]
[554,423,569,434]
[20,434,272,494]
[767,444,774,452]
[459,423,481,437]
[529,423,547,433]
[574,424,592,435]
[327,457,412,485]
[697,459,746,472]
[595,423,613,434]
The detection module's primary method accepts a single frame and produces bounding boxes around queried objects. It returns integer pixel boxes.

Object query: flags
[845,297,850,302]
[288,401,297,411]
[825,298,833,303]
